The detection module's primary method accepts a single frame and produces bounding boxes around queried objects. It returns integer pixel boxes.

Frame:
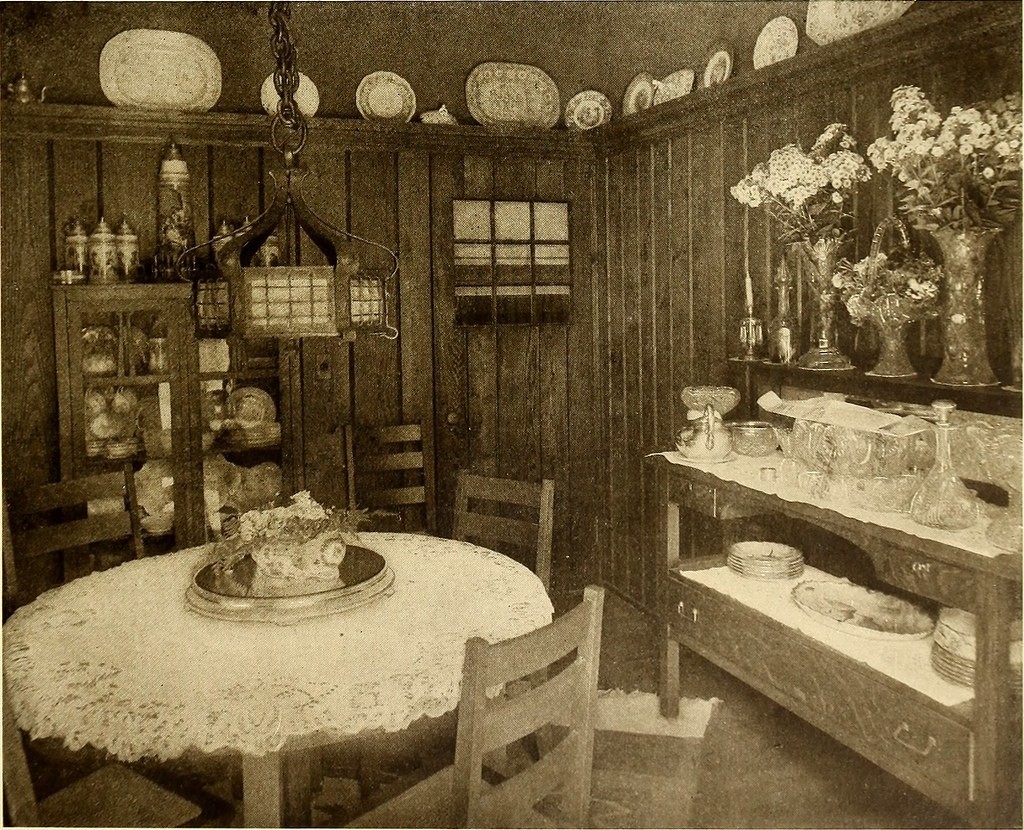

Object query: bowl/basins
[728,417,778,457]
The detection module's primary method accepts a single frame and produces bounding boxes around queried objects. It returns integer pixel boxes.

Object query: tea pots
[674,407,733,462]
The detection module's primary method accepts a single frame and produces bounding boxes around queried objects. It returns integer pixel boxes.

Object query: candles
[743,273,753,307]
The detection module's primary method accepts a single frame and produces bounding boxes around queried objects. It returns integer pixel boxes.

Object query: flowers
[728,82,1024,327]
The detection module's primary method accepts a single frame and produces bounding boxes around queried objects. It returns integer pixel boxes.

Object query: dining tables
[5,526,555,827]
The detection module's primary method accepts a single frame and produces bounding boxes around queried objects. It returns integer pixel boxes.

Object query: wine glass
[785,418,974,509]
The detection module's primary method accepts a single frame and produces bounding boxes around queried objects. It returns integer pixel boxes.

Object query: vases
[932,221,1006,386]
[793,236,857,372]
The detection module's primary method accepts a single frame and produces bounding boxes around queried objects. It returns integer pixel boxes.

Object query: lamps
[177,2,399,341]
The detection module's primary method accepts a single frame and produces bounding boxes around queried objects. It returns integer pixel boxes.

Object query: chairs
[4,697,204,826]
[344,423,612,829]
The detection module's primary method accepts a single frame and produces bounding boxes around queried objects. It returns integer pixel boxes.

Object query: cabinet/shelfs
[651,453,1023,829]
[56,288,301,546]
[452,195,572,330]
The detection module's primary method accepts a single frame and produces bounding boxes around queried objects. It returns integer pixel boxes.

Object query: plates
[724,540,806,579]
[220,386,281,447]
[85,385,160,438]
[929,605,1023,695]
[82,324,148,376]
[87,462,281,538]
[791,579,934,639]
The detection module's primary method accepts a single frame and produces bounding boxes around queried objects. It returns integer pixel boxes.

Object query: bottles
[146,255,202,281]
[909,397,978,529]
[210,216,280,271]
[65,218,139,286]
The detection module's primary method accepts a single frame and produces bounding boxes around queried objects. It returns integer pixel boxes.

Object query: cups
[86,436,141,462]
[50,270,81,285]
[144,429,215,456]
